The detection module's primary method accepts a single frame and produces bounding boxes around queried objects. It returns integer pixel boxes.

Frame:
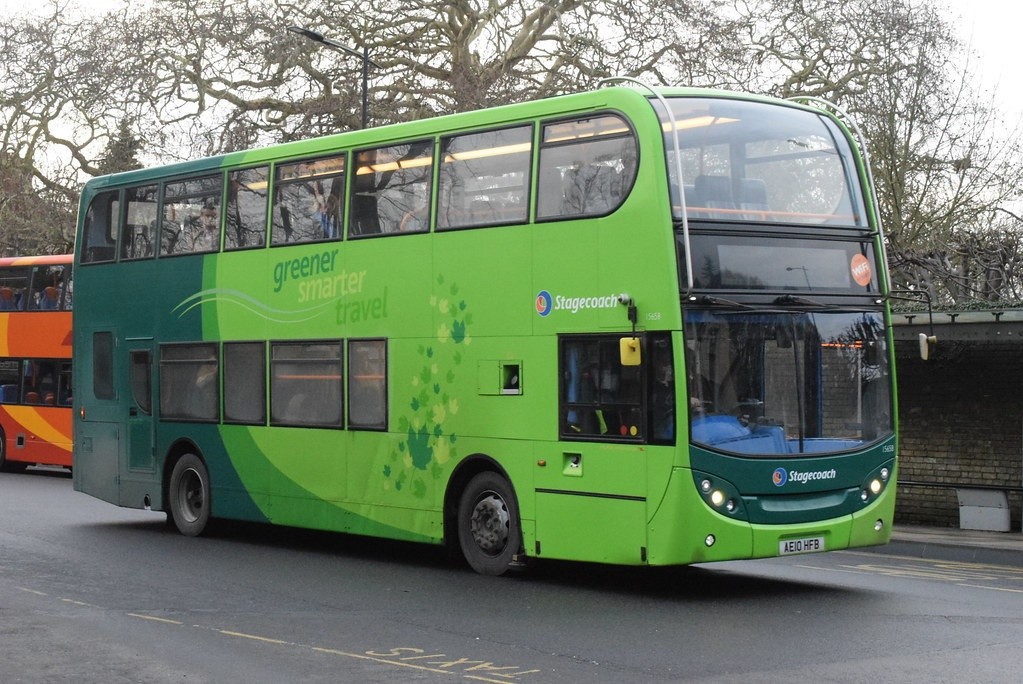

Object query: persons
[629,359,709,442]
[177,206,231,252]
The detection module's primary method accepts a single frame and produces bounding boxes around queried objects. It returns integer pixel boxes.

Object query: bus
[71,77,938,579]
[0,253,74,473]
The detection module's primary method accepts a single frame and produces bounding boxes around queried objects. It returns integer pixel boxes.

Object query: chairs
[526,164,774,220]
[86,215,202,263]
[0,385,73,406]
[0,287,59,309]
